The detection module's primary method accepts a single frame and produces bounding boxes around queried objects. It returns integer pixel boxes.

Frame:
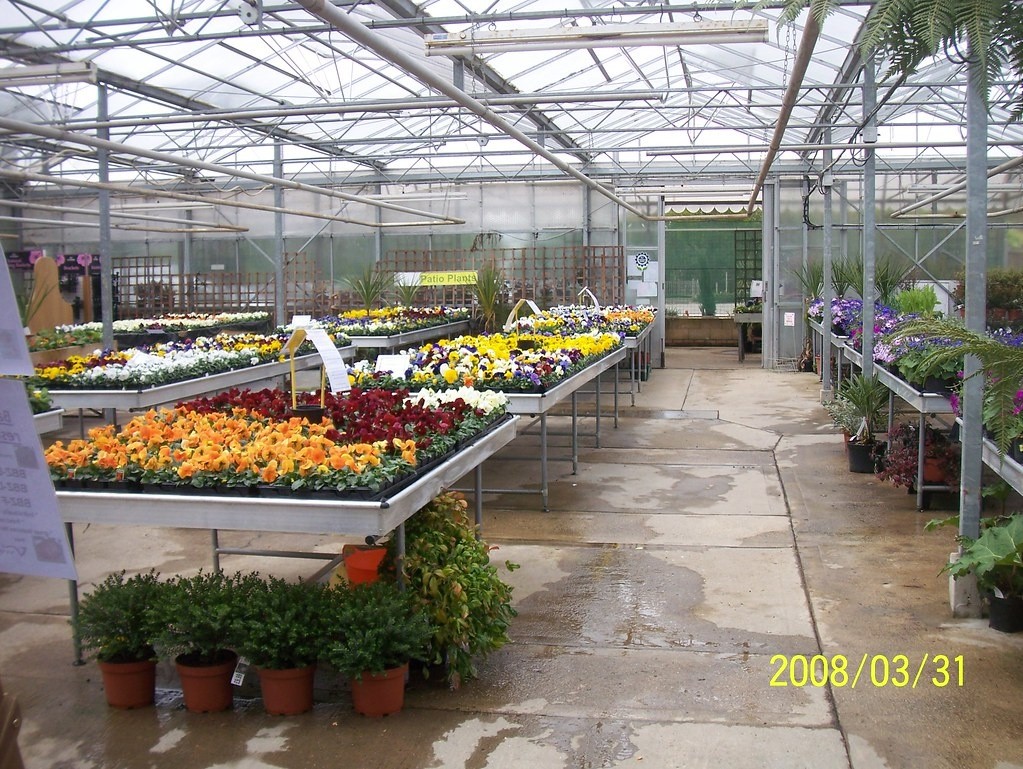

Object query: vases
[924,457,947,482]
[815,357,836,376]
[1013,438,1023,464]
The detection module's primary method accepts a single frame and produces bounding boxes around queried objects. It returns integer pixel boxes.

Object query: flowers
[0,304,1023,505]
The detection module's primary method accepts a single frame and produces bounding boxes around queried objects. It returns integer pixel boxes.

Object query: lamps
[423,7,770,57]
[0,58,97,89]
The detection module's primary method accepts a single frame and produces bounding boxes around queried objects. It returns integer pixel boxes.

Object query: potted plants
[140,566,252,714]
[896,343,964,394]
[922,480,1023,632]
[67,565,176,710]
[948,261,1023,324]
[834,371,889,474]
[317,574,441,718]
[228,569,333,717]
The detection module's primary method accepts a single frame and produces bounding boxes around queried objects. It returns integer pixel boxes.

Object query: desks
[733,312,762,364]
